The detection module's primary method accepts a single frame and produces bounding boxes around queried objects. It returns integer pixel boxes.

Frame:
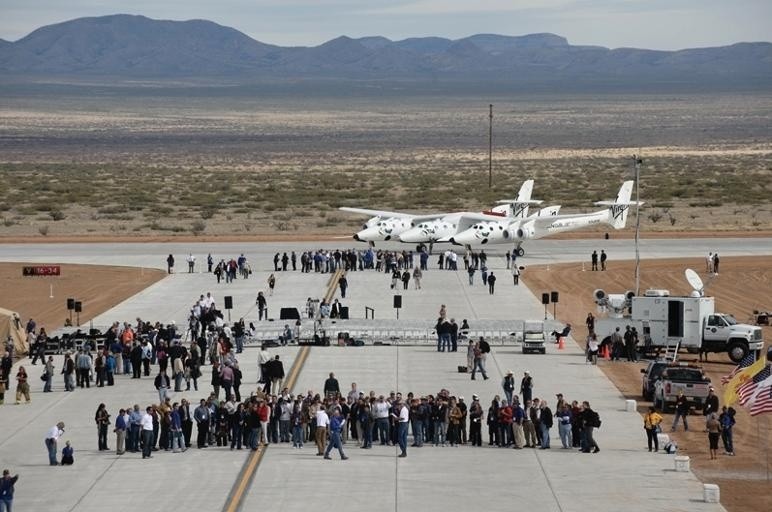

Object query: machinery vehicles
[522,319,548,354]
[593,267,764,363]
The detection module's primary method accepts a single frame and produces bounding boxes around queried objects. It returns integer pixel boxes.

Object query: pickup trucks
[654,364,713,416]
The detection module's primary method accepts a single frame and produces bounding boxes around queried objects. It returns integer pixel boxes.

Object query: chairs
[31,316,572,355]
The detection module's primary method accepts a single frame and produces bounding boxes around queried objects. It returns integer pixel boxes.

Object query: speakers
[67,299,75,309]
[224,296,232,309]
[394,295,402,308]
[75,301,82,312]
[542,293,550,304]
[551,292,559,302]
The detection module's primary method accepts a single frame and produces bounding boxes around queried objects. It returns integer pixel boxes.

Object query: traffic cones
[558,338,564,350]
[604,343,610,359]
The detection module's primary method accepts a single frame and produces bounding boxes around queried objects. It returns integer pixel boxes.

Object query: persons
[166,251,252,285]
[462,247,498,293]
[44,398,194,467]
[644,385,738,459]
[151,292,291,403]
[707,251,720,273]
[591,248,608,270]
[552,311,642,363]
[1,311,182,405]
[484,369,603,452]
[505,249,521,286]
[388,248,457,291]
[273,250,414,274]
[1,468,20,511]
[432,305,490,380]
[194,371,485,459]
[255,273,348,320]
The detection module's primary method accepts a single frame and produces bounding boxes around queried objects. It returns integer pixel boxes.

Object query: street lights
[631,151,645,298]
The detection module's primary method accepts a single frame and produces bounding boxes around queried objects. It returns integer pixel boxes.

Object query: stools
[590,355,598,364]
[703,482,720,503]
[624,398,639,414]
[650,432,671,450]
[673,455,692,473]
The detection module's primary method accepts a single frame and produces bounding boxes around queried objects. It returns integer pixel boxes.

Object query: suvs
[638,360,681,402]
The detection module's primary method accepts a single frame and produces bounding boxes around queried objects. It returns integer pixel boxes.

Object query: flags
[718,345,771,417]
